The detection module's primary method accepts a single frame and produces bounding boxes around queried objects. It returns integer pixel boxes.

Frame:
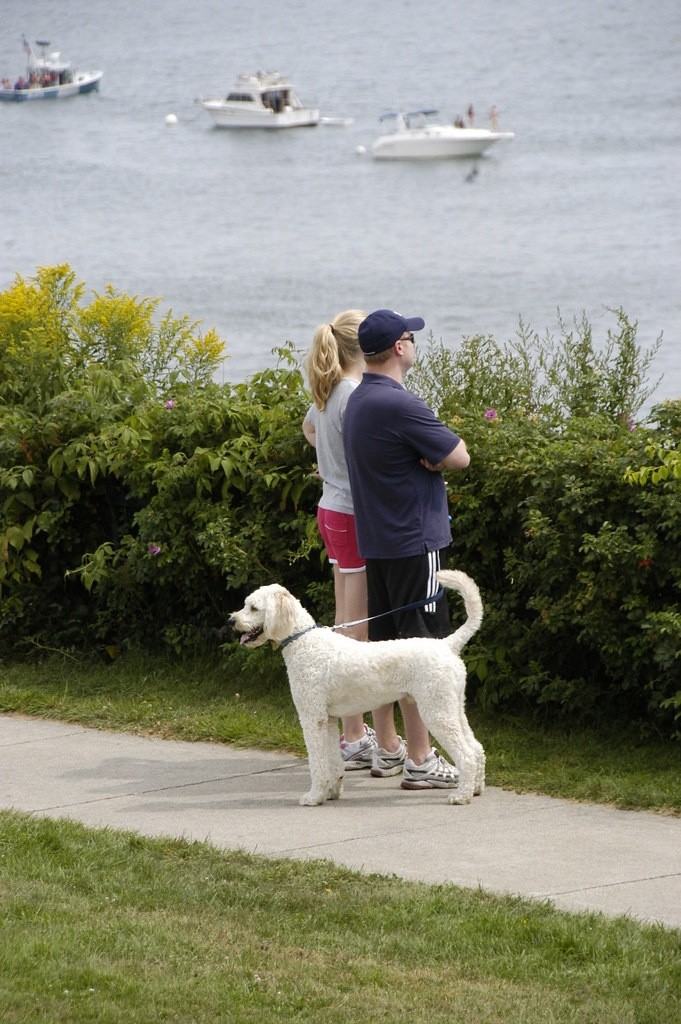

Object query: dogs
[228,570,487,806]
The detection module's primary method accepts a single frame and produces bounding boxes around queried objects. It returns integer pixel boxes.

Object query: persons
[343,310,471,790]
[301,311,377,769]
[2,72,58,89]
[455,102,499,133]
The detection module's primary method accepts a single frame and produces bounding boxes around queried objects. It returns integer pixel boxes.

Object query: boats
[0,37,103,103]
[369,108,512,160]
[197,68,320,131]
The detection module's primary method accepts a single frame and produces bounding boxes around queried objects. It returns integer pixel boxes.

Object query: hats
[358,309,425,356]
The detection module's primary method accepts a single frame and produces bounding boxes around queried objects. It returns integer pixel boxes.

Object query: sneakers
[401,747,459,790]
[371,735,408,777]
[339,723,377,770]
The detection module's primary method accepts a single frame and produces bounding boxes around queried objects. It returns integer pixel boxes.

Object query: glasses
[398,334,414,344]
